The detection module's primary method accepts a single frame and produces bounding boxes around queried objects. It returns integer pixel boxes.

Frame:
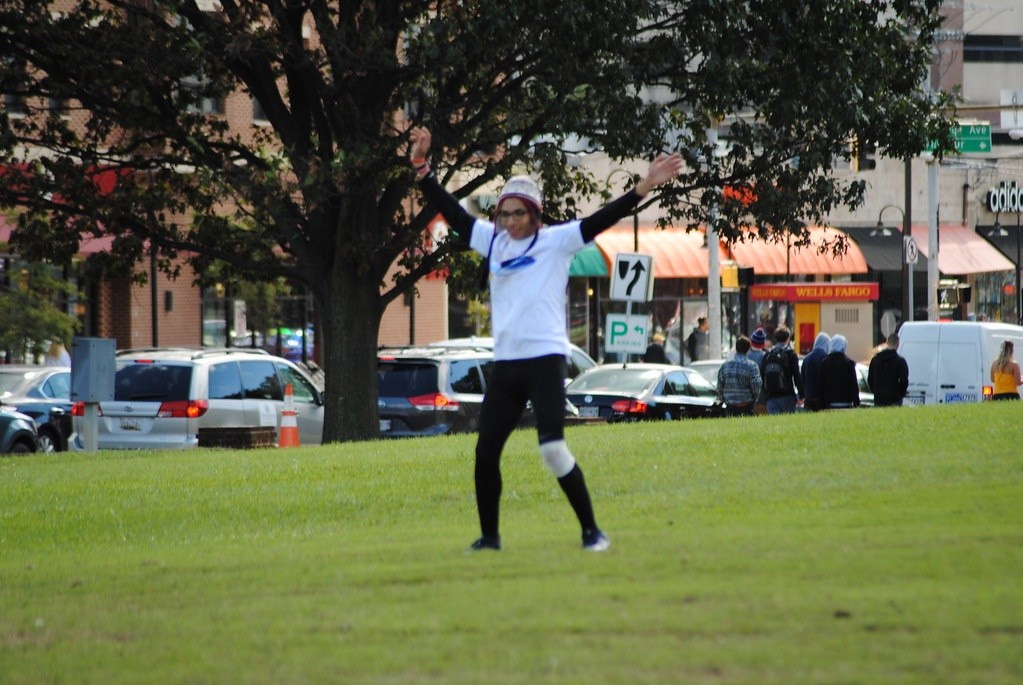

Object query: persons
[718,334,763,416]
[978,312,988,321]
[760,325,805,416]
[991,340,1023,402]
[44,339,72,367]
[641,330,671,365]
[747,326,769,371]
[867,334,911,408]
[819,332,862,409]
[406,124,684,553]
[688,317,710,362]
[916,309,929,321]
[801,332,831,411]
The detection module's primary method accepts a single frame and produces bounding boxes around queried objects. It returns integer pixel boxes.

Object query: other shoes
[470,539,500,552]
[583,531,609,551]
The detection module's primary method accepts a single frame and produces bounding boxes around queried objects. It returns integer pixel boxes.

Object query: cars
[565,362,727,424]
[688,359,728,390]
[0,360,74,457]
[791,355,915,413]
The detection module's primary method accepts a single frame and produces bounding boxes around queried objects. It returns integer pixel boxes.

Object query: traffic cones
[275,383,303,448]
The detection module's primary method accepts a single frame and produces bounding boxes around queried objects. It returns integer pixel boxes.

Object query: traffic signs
[604,312,649,356]
[921,120,992,155]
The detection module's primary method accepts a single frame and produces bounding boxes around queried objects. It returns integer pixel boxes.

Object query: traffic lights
[937,285,958,306]
[848,129,878,175]
[958,282,973,305]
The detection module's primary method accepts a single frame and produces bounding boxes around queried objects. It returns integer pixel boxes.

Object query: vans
[895,319,1023,406]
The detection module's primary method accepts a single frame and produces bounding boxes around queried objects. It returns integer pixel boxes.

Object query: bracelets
[412,164,426,171]
[410,159,426,163]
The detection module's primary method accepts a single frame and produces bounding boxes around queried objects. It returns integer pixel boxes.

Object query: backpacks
[763,351,788,393]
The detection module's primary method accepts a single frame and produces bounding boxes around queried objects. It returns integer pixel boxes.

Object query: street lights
[871,203,908,321]
[599,167,640,362]
[988,204,1022,326]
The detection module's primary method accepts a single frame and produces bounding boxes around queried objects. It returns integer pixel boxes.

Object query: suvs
[66,345,326,451]
[375,344,581,440]
[428,334,599,388]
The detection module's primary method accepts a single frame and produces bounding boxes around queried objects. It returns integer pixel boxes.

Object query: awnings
[898,220,1016,276]
[974,222,1023,269]
[0,212,203,264]
[593,220,869,277]
[569,241,608,277]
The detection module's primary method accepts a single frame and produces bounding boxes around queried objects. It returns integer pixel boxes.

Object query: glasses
[499,208,531,222]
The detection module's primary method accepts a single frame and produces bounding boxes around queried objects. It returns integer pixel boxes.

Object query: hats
[479,175,543,229]
[750,328,766,350]
